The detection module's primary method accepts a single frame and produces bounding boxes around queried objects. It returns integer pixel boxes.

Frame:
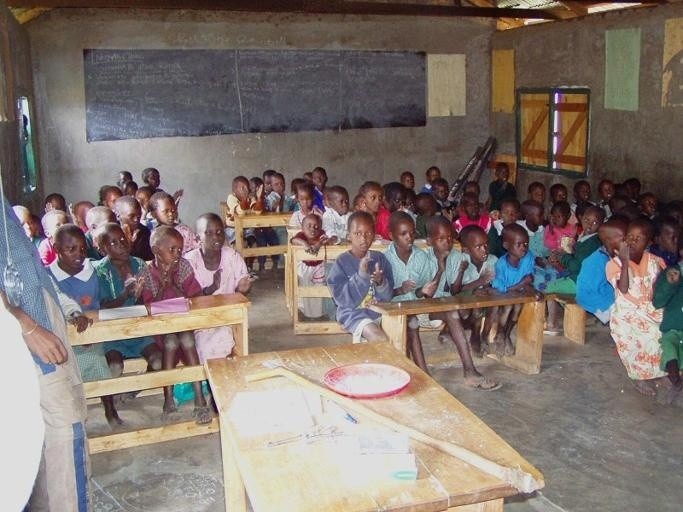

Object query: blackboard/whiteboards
[83,48,427,142]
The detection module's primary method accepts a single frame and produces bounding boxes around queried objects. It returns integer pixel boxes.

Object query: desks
[202,343,545,511]
[219,203,298,274]
[64,259,252,456]
[285,234,589,378]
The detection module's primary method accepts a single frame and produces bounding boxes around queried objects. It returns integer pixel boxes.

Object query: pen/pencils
[331,400,357,423]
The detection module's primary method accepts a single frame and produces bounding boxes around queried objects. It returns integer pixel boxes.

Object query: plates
[324,362,408,398]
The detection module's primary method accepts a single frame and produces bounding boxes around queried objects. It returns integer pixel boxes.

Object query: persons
[0,187,90,512]
[297,166,682,396]
[225,167,329,281]
[13,167,251,431]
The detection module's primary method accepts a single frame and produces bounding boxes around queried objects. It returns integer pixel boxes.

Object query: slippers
[460,375,504,391]
[160,407,182,425]
[543,325,562,336]
[625,372,657,397]
[191,405,213,425]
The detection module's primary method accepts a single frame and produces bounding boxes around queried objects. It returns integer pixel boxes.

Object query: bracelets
[22,323,38,335]
